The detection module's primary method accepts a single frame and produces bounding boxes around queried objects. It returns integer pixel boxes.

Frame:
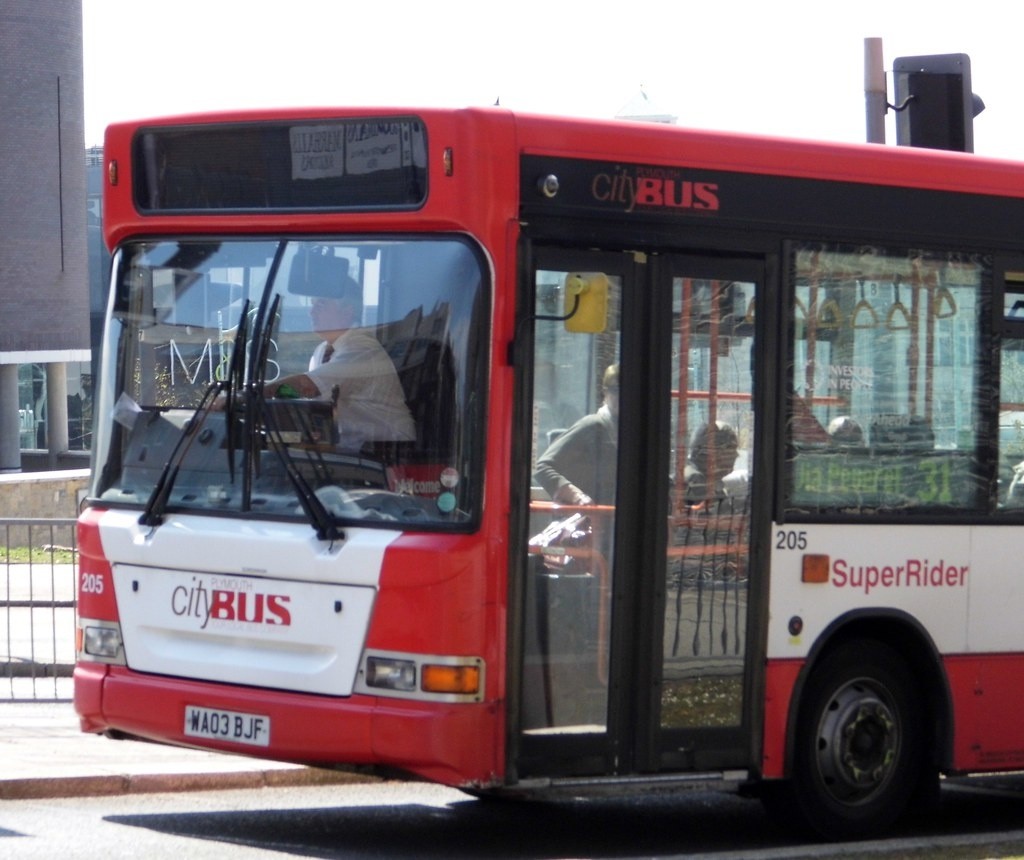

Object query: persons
[207,277,421,466]
[532,360,1024,560]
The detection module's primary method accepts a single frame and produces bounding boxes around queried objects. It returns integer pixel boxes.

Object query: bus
[75,106,1021,847]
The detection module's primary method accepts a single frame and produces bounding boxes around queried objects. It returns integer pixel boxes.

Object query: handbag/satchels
[528,512,590,573]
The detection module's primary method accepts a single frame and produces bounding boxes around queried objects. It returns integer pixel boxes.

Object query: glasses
[604,385,620,396]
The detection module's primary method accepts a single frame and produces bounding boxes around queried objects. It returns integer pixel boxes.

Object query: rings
[212,402,216,406]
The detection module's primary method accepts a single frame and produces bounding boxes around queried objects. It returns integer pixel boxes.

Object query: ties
[321,345,340,408]
